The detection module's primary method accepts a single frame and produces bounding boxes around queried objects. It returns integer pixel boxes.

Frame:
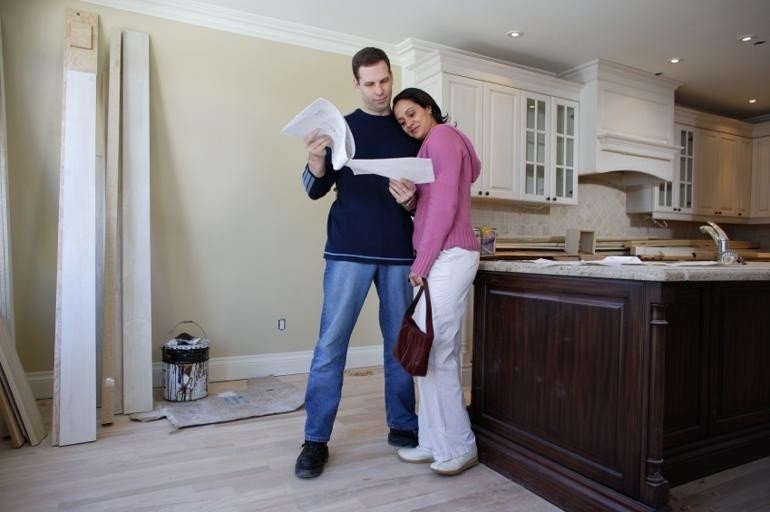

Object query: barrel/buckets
[160,320,210,402]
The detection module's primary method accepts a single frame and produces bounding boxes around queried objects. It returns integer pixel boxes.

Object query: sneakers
[386,429,418,446]
[430,448,480,476]
[295,442,327,477]
[396,446,431,463]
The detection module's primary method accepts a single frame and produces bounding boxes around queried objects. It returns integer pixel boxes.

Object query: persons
[393,89,481,476]
[295,48,420,480]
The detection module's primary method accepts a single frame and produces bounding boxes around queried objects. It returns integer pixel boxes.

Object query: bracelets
[401,196,417,208]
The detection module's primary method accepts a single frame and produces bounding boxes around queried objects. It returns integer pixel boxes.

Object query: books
[282,96,435,187]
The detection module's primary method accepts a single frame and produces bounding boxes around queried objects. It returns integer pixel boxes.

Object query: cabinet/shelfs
[520,88,581,208]
[626,119,693,222]
[694,124,751,225]
[751,134,769,225]
[408,69,522,205]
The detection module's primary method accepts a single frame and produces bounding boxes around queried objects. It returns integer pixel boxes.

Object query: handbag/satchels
[393,313,433,377]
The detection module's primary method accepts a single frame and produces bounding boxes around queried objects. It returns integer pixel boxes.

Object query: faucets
[698,220,729,253]
